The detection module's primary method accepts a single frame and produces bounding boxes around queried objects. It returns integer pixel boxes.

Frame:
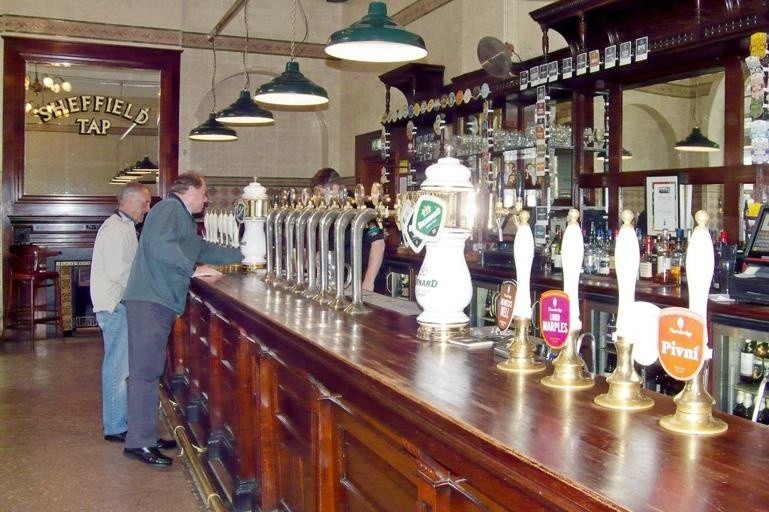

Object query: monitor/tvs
[746,203,769,262]
[502,206,536,235]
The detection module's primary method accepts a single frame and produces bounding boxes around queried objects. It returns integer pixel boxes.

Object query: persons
[309,169,387,296]
[120,170,249,467]
[89,180,151,441]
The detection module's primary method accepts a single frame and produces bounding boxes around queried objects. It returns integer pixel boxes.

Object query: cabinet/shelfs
[582,292,685,398]
[378,264,414,304]
[710,316,769,421]
[473,281,578,380]
[387,90,573,251]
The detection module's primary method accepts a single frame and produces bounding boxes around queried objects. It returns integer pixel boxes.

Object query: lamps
[255,0,329,106]
[324,1,429,63]
[674,75,720,152]
[595,142,636,161]
[24,61,73,114]
[214,2,273,125]
[188,39,239,141]
[109,132,158,185]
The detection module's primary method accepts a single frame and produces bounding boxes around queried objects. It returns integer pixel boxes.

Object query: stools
[0,271,63,352]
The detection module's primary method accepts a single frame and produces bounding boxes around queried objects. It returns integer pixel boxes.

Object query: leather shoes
[124,446,172,468]
[156,439,176,451]
[105,431,127,442]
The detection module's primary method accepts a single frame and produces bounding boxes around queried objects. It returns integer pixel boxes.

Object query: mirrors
[1,35,184,222]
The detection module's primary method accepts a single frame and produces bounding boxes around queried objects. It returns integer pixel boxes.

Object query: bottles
[540,215,733,296]
[600,312,769,425]
